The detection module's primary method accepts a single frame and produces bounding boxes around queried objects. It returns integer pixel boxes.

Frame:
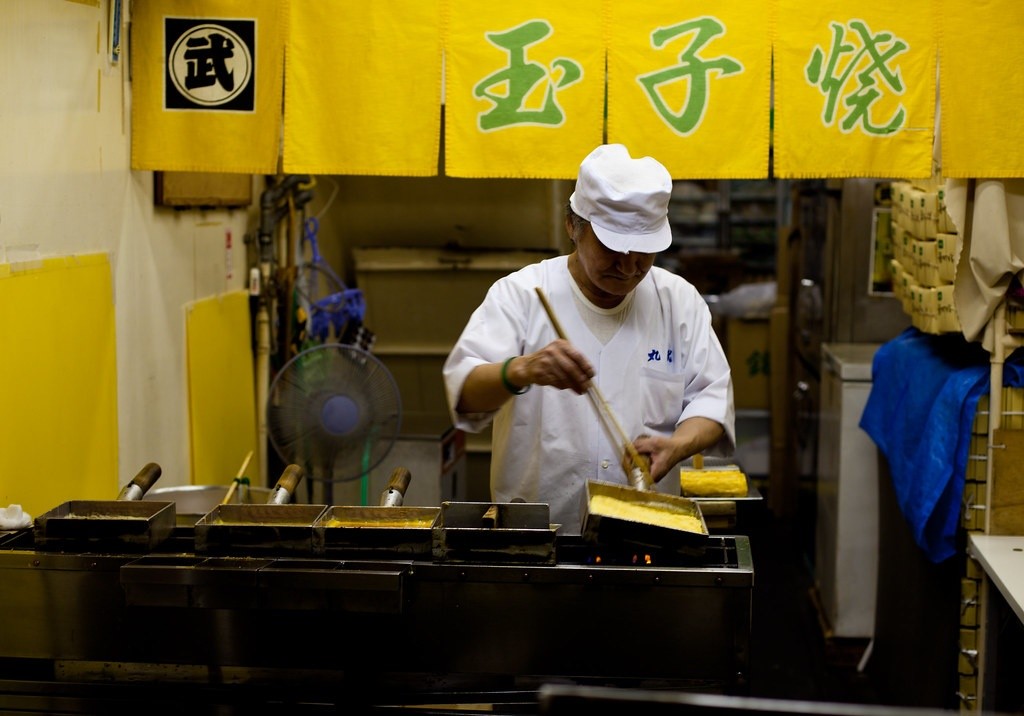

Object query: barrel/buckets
[141,484,273,525]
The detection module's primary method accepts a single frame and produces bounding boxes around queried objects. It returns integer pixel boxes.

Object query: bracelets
[502,356,531,395]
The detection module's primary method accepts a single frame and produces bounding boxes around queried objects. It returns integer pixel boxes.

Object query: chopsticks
[533,284,658,487]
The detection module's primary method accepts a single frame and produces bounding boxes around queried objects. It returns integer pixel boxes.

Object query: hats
[570,143,673,255]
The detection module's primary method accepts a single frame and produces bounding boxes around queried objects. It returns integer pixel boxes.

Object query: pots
[33,462,176,550]
[440,497,562,565]
[194,463,327,558]
[578,434,709,562]
[313,467,441,562]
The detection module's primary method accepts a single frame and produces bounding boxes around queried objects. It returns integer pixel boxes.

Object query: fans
[264,343,402,507]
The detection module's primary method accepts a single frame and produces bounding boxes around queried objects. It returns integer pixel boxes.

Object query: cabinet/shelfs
[353,246,553,452]
[667,179,777,299]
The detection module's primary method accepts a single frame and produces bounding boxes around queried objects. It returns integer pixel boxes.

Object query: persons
[441,143,738,533]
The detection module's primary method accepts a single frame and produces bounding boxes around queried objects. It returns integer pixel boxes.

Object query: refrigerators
[814,342,884,639]
